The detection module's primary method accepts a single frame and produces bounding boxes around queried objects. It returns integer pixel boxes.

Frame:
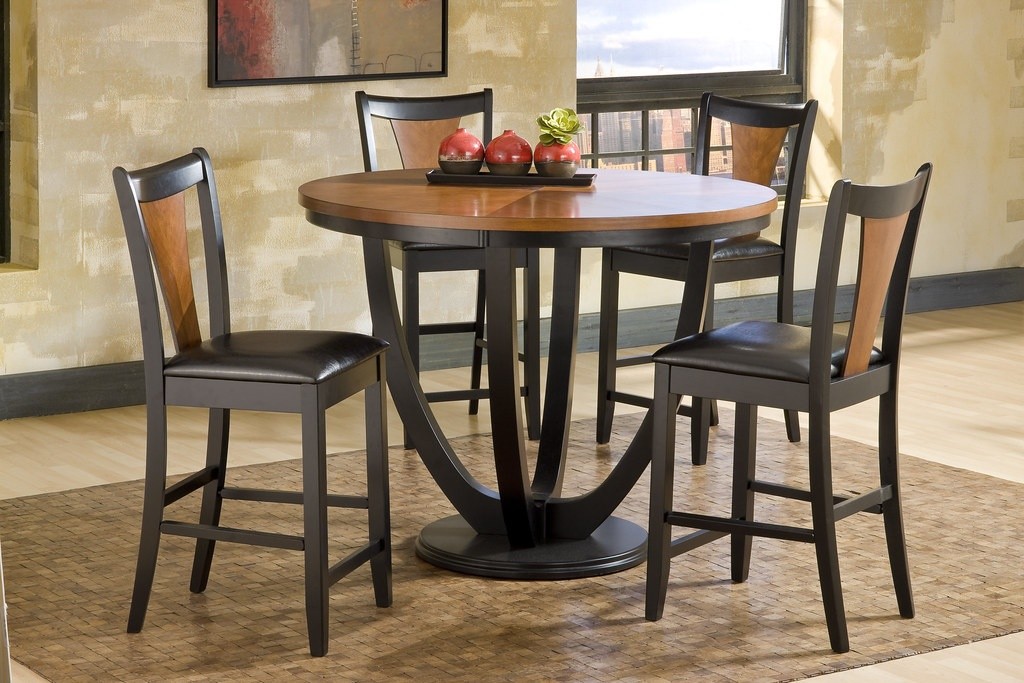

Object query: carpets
[0,405,1024,683]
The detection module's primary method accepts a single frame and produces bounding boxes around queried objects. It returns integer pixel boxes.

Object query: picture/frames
[205,0,450,89]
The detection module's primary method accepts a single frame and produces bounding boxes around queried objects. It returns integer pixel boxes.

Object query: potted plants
[534,108,582,177]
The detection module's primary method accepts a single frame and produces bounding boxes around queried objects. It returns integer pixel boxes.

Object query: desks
[300,170,780,579]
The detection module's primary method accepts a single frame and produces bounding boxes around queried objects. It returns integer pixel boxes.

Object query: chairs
[111,146,393,659]
[647,159,936,650]
[596,86,820,470]
[358,88,538,444]
